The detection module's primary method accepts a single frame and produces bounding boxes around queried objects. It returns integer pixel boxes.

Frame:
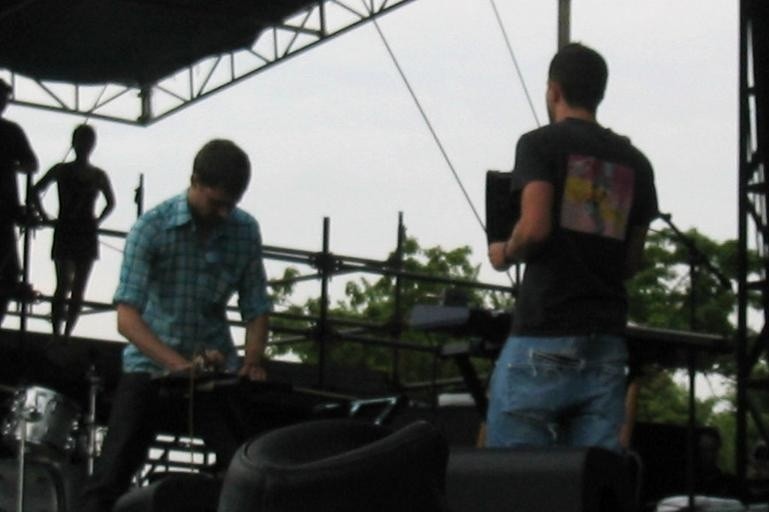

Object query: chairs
[217,418,449,512]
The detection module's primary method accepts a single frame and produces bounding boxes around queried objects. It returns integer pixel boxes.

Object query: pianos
[155,366,484,448]
[409,304,728,355]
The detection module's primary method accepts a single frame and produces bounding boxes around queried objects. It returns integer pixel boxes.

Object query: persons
[68,135,276,512]
[28,124,117,336]
[0,77,40,333]
[487,41,660,450]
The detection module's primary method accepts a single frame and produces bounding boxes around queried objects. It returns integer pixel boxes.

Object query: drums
[67,426,108,459]
[0,462,65,511]
[1,385,82,451]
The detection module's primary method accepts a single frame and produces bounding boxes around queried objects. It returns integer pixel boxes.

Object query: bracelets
[503,238,513,267]
